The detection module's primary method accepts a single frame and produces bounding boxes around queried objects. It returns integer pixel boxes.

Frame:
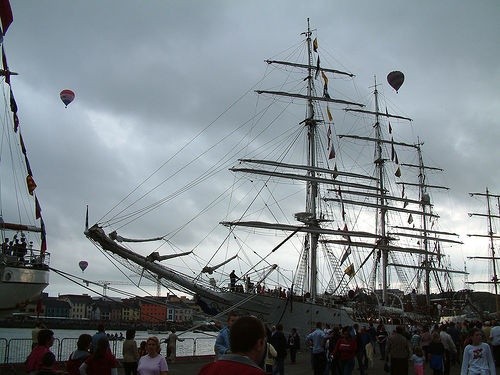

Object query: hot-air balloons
[60,89,75,109]
[79,260,89,273]
[387,70,404,93]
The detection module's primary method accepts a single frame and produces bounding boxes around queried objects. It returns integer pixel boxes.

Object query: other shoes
[380,358,385,360]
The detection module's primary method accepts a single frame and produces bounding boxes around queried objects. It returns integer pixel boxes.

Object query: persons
[0,237,500,375]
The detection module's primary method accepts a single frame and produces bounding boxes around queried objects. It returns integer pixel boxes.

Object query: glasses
[52,336,55,340]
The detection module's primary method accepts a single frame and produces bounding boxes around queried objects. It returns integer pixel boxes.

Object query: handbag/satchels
[384,362,390,373]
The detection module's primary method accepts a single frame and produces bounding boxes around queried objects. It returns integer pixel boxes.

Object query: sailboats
[0,1,53,314]
[85,0,500,356]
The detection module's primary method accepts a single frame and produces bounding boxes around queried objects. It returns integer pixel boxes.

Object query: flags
[408,213,413,224]
[344,262,356,278]
[395,167,401,178]
[313,37,318,52]
